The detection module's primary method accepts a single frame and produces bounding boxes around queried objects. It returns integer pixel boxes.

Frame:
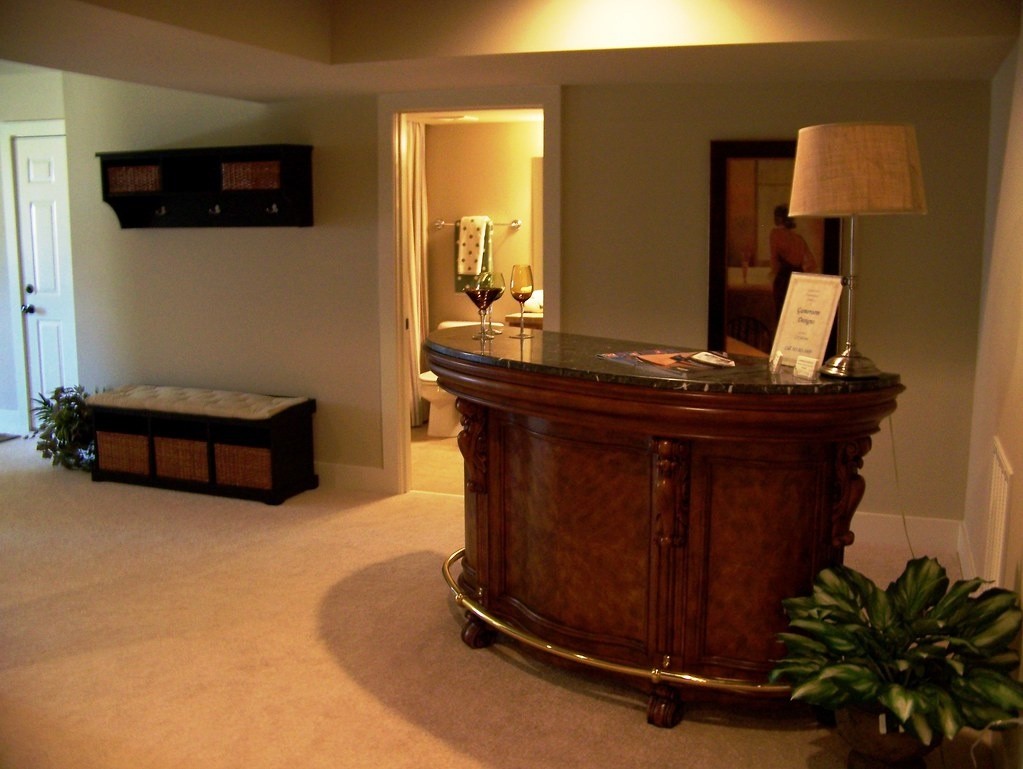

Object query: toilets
[417,317,503,440]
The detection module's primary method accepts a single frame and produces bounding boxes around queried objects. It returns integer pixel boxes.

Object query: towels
[457,214,492,277]
[453,219,494,299]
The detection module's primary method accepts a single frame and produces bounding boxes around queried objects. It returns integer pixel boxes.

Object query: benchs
[88,383,321,507]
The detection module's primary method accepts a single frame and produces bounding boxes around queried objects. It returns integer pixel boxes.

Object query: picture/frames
[706,139,842,367]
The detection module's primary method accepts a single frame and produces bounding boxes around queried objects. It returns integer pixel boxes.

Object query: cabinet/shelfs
[95,143,314,227]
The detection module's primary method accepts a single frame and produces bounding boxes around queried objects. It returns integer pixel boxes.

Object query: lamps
[787,122,928,380]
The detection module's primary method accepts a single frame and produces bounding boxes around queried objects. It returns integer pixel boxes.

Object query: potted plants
[22,383,106,474]
[770,554,1023,766]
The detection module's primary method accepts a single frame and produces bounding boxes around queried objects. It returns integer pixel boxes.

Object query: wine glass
[464,273,502,341]
[478,272,506,336]
[509,264,535,339]
